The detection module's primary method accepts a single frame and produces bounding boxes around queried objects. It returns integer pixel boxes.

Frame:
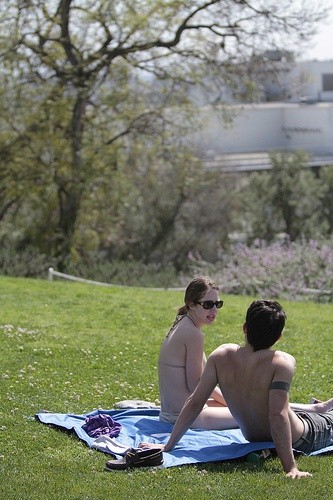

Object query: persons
[138,300,333,480]
[158,277,333,430]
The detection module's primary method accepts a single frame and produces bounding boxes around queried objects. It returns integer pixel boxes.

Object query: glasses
[196,300,223,310]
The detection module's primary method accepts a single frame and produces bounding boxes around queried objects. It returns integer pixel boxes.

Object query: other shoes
[104,449,163,472]
